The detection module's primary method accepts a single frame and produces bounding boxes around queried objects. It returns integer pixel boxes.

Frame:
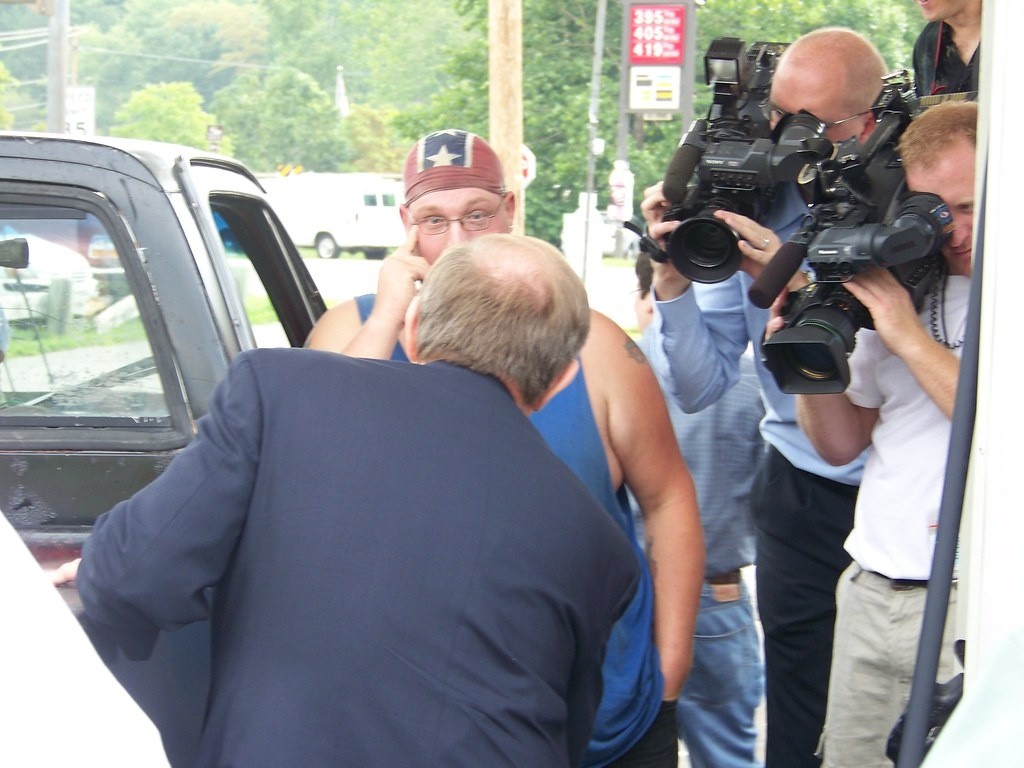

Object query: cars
[2,221,98,319]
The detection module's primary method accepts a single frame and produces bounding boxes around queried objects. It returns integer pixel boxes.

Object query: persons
[628,26,890,768]
[907,0,982,112]
[49,232,641,768]
[761,98,977,768]
[634,289,764,768]
[300,128,704,768]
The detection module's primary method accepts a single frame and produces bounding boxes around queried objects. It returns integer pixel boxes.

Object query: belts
[868,571,929,591]
[704,569,742,584]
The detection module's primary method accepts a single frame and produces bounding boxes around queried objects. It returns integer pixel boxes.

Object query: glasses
[408,197,504,235]
[758,97,870,128]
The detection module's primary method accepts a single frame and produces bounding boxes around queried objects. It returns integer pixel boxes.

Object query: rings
[762,238,770,246]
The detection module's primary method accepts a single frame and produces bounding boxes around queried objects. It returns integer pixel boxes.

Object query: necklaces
[942,271,948,343]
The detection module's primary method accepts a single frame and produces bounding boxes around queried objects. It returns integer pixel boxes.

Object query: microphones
[661,119,708,204]
[748,230,811,310]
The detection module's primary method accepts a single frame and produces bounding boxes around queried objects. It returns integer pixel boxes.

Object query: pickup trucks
[0,132,327,665]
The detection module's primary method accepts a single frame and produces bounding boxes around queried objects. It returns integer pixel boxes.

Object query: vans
[262,172,404,260]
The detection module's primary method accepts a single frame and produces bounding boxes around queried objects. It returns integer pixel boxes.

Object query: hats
[403,129,506,208]
